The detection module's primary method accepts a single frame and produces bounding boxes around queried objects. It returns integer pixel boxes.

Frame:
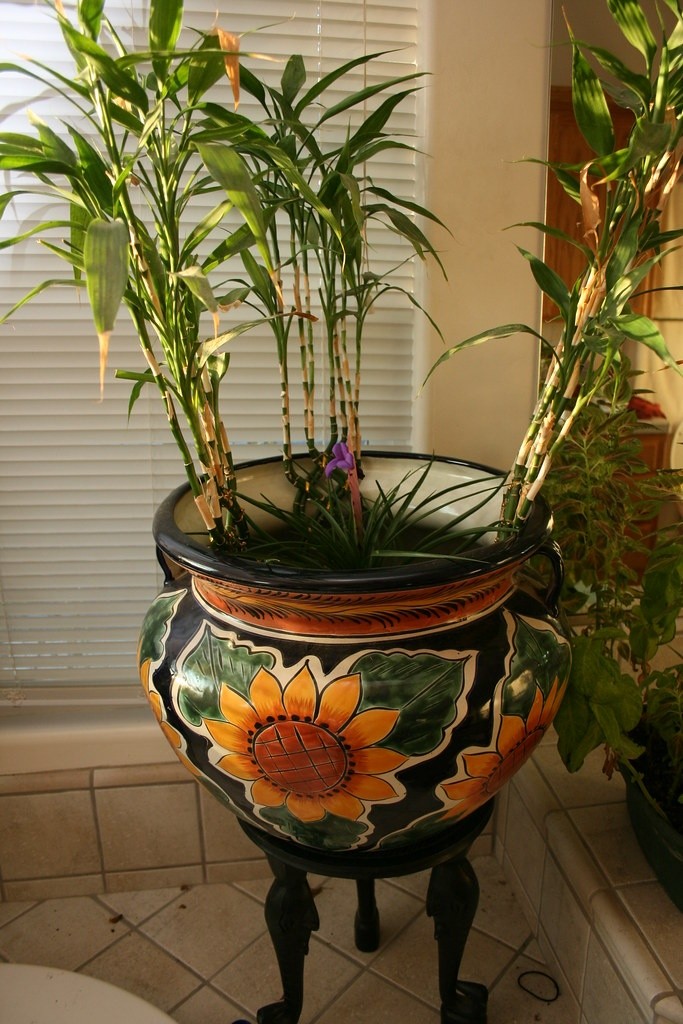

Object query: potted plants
[0,0,683,1024]
[611,665,683,915]
